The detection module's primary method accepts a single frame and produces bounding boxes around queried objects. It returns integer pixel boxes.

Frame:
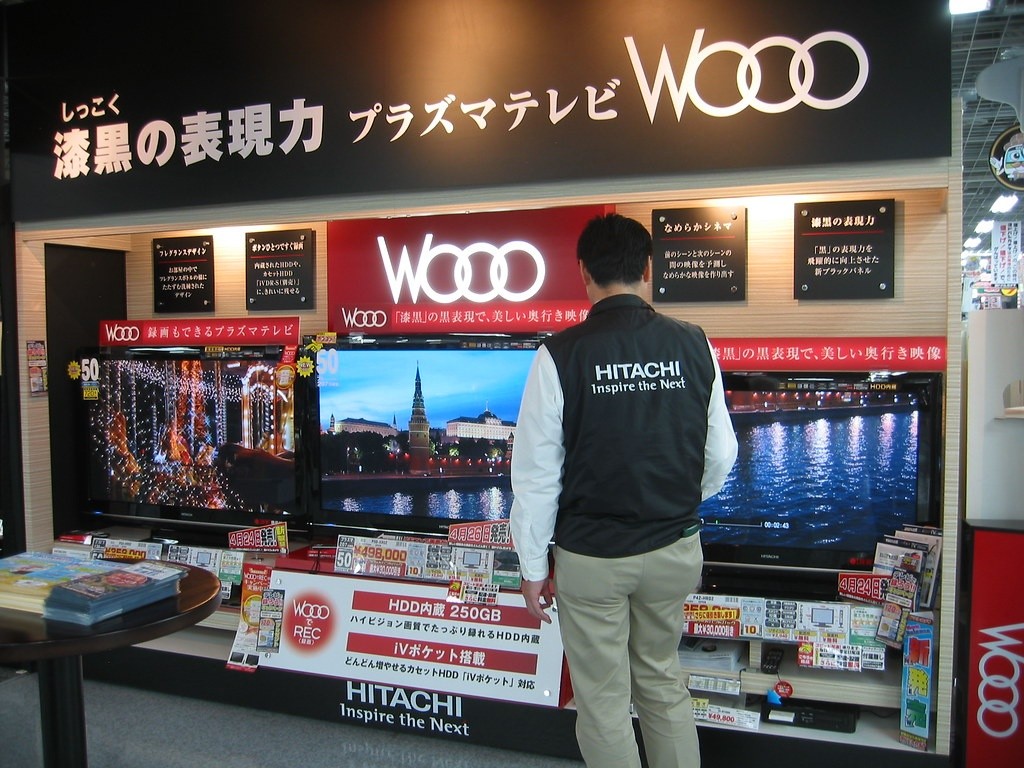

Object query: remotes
[762,648,785,674]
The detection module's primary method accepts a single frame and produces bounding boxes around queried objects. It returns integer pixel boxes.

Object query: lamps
[989,193,1019,214]
[974,219,995,234]
[962,237,981,259]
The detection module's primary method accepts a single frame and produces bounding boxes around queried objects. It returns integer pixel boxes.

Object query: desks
[0,555,222,768]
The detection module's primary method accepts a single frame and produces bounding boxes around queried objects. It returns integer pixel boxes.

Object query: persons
[509,212,739,768]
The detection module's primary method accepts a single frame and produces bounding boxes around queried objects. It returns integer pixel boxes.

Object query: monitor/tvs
[303,335,561,543]
[246,655,259,665]
[812,608,835,627]
[695,371,945,601]
[229,652,244,662]
[196,552,212,568]
[75,344,307,547]
[463,551,481,569]
